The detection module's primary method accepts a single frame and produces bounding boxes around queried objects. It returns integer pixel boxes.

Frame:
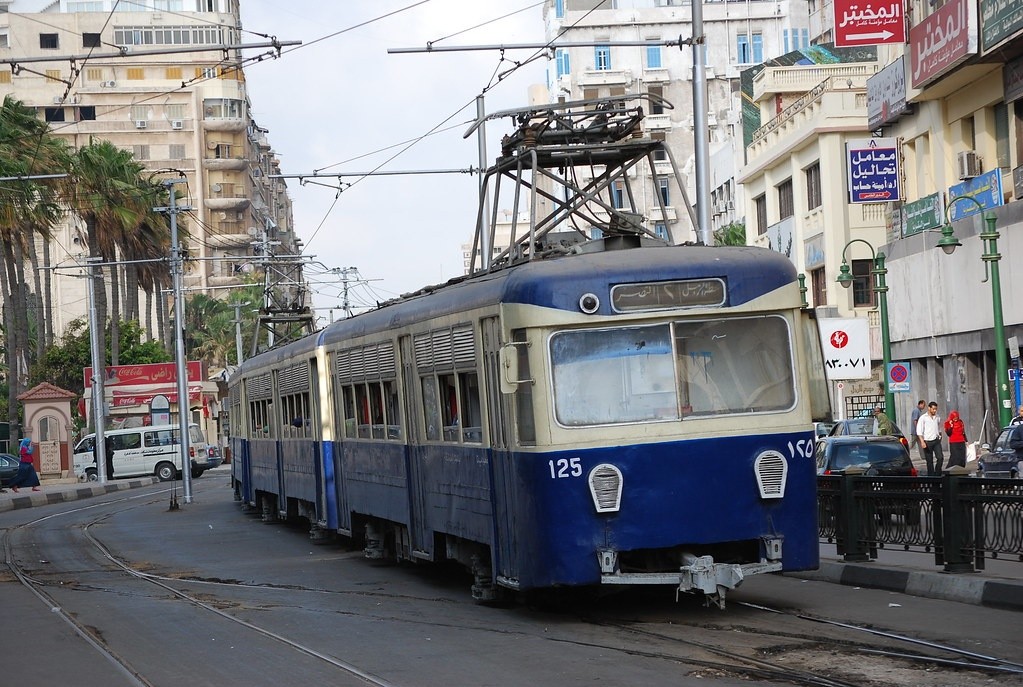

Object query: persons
[876,412,892,436]
[9,438,41,492]
[945,412,968,468]
[1009,424,1023,490]
[873,407,882,436]
[916,404,944,476]
[909,400,926,463]
[93,436,115,480]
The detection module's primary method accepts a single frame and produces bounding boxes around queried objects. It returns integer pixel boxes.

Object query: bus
[225,211,835,607]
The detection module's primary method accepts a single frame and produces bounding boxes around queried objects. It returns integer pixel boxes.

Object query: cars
[813,422,839,443]
[154,437,222,470]
[855,407,885,419]
[0,453,21,486]
[815,432,920,529]
[978,424,1023,490]
[818,418,911,457]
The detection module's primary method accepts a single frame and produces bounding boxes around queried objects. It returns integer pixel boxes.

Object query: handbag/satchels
[936,435,942,443]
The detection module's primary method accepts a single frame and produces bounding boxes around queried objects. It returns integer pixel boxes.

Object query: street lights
[235,310,260,367]
[935,195,1013,426]
[837,240,898,428]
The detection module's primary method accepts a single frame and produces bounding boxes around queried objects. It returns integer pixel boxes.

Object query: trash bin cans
[827,464,879,563]
[930,464,986,577]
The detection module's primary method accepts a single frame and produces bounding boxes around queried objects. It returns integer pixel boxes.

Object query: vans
[72,423,211,480]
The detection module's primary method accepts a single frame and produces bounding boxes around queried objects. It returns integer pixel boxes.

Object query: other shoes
[32,488,40,491]
[12,487,19,493]
